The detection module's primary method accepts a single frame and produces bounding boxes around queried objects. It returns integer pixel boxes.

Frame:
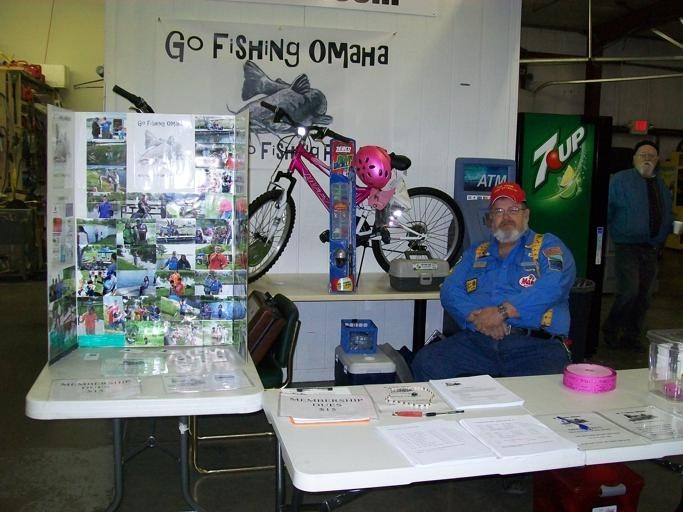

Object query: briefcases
[246,290,286,369]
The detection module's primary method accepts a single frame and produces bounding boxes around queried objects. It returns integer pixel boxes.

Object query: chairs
[191,294,301,474]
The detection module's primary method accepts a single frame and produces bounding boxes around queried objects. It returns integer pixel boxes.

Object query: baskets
[340,318,378,353]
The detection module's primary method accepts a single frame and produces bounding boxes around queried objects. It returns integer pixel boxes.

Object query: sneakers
[623,340,645,353]
[599,325,620,349]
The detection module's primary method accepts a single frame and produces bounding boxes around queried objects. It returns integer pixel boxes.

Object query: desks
[264,369,683,512]
[248,272,445,364]
[24,345,265,512]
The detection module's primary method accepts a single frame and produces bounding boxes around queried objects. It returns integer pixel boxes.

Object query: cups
[673,220,683,235]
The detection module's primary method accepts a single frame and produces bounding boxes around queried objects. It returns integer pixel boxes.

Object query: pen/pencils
[425,409,465,416]
[557,416,591,431]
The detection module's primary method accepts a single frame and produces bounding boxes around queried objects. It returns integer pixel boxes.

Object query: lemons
[559,166,574,187]
[560,182,578,199]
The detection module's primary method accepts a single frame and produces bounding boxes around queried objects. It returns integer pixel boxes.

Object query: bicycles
[248,99,469,285]
[112,83,155,113]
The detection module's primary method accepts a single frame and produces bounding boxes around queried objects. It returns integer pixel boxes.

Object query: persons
[599,139,676,355]
[409,179,578,384]
[49,107,248,360]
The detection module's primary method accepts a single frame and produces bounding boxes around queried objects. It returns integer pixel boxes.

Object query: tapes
[562,363,617,393]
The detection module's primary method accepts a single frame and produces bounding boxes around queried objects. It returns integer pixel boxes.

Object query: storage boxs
[388,259,450,292]
[646,328,683,402]
[334,345,397,386]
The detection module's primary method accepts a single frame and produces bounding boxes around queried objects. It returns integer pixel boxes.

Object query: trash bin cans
[568,278,596,364]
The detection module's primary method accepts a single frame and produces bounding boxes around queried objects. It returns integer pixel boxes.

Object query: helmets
[355,146,391,189]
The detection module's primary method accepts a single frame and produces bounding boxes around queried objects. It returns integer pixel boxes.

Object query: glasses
[494,207,525,215]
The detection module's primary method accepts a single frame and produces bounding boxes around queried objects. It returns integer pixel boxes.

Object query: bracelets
[495,303,509,321]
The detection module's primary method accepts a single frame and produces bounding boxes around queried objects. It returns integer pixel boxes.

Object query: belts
[506,326,562,340]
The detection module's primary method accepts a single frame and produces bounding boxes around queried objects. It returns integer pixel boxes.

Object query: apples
[546,151,562,168]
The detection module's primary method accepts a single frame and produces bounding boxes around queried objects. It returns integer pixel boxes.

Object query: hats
[491,181,526,206]
[634,140,658,155]
